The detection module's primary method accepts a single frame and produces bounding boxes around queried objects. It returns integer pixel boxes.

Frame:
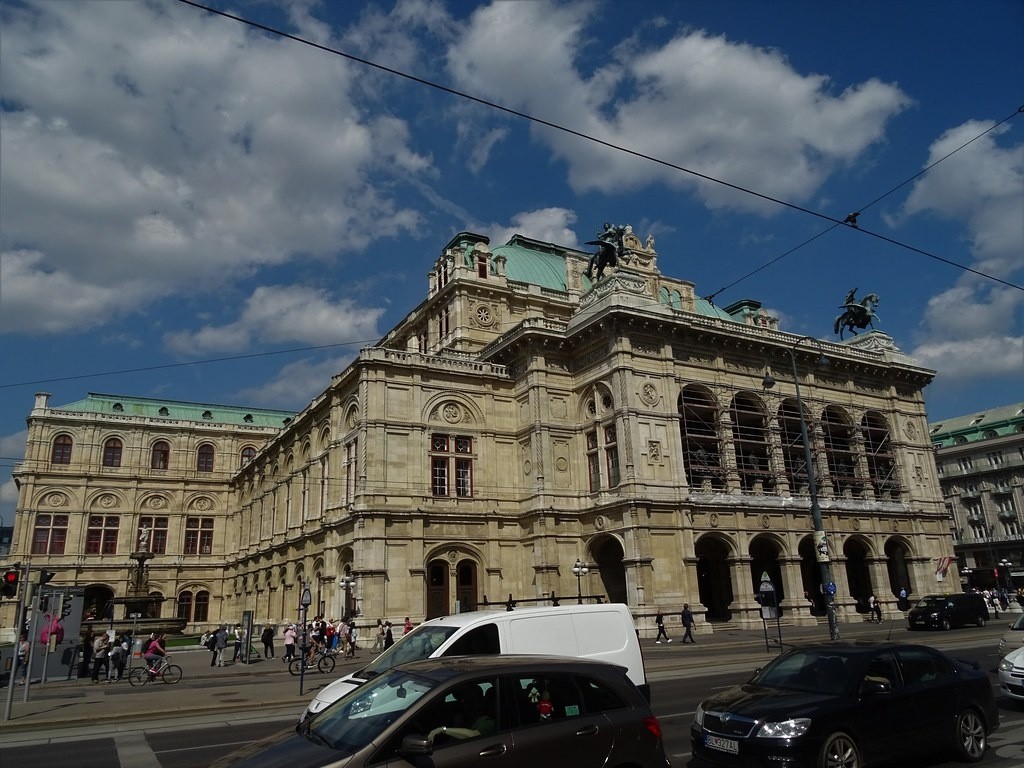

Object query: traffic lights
[2,570,21,599]
[58,595,72,619]
[35,570,56,585]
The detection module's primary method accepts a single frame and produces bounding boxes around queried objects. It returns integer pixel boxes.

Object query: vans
[908,593,990,631]
[301,603,650,721]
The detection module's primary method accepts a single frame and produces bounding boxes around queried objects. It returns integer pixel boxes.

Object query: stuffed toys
[528,687,554,719]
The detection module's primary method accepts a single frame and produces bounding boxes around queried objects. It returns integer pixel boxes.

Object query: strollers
[237,644,261,659]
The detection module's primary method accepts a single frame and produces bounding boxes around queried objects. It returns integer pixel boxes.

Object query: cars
[690,641,1000,768]
[998,638,1024,700]
[998,613,1024,659]
[207,654,673,768]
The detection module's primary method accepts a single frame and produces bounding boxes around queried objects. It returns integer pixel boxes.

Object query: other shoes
[93,680,98,683]
[19,682,24,687]
[115,679,118,681]
[150,667,158,674]
[104,679,110,683]
[4,685,8,688]
[656,641,661,643]
[308,664,317,668]
[667,639,672,643]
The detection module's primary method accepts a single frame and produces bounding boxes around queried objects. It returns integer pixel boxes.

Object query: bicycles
[129,655,182,686]
[288,642,335,676]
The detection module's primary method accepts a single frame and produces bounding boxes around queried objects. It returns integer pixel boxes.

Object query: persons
[79,625,133,684]
[306,615,358,669]
[597,222,617,242]
[846,287,859,304]
[129,565,138,586]
[139,524,149,542]
[680,604,697,644]
[864,675,892,691]
[900,587,907,611]
[969,587,1024,614]
[200,622,277,667]
[143,633,169,676]
[142,564,151,586]
[870,594,884,625]
[655,609,672,644]
[370,617,414,654]
[3,629,30,689]
[426,716,496,745]
[282,624,298,663]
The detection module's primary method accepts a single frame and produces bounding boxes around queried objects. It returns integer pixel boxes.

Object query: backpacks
[346,631,351,642]
[141,638,156,654]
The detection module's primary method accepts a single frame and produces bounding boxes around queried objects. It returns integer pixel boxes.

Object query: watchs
[442,726,446,733]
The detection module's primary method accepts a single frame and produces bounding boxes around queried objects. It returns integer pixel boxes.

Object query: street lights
[961,567,976,594]
[339,576,356,617]
[999,559,1012,592]
[762,337,841,639]
